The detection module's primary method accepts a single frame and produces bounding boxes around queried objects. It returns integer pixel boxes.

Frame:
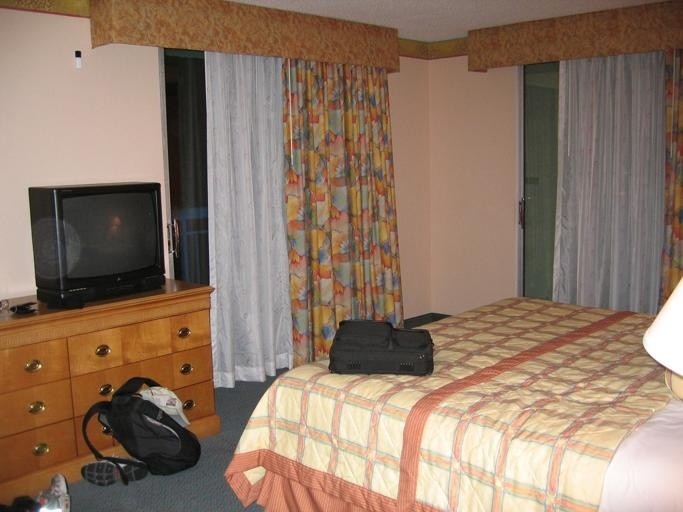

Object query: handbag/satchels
[328,320,434,376]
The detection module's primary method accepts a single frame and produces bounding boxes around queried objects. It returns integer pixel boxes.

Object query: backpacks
[81,377,200,485]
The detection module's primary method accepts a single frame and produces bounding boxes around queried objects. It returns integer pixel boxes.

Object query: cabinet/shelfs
[0,277,222,506]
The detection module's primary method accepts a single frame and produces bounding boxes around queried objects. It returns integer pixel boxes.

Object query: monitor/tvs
[28,182,166,310]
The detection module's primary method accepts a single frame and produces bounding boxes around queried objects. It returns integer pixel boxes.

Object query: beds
[224,297,683,511]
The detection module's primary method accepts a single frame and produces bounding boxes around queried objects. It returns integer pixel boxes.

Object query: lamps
[642,276,683,378]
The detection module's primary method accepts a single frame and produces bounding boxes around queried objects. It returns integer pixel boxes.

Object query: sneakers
[80,456,147,487]
[34,473,71,512]
